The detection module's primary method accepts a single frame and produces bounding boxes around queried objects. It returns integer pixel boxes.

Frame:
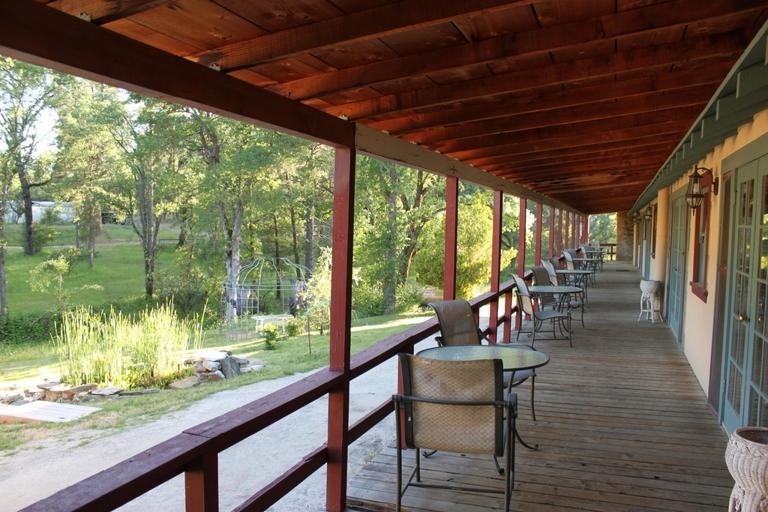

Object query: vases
[725,426,768,512]
[639,280,665,323]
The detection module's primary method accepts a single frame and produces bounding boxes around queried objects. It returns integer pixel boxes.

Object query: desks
[252,315,294,334]
[554,268,594,302]
[415,345,552,438]
[526,285,583,336]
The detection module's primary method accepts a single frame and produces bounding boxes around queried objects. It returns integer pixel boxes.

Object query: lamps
[632,202,656,224]
[686,165,718,216]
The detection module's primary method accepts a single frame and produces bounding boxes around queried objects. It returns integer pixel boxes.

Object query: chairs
[558,246,605,298]
[539,258,586,327]
[418,300,540,423]
[397,350,540,510]
[511,272,573,349]
